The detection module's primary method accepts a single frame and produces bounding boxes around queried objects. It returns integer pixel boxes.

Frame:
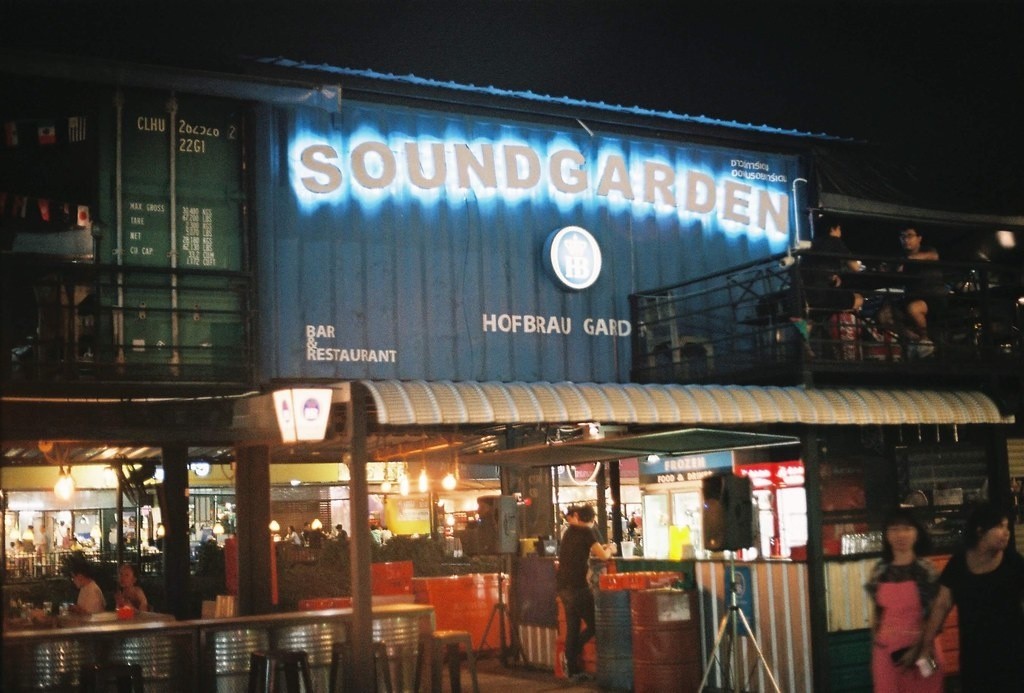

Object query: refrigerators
[638,449,737,560]
[734,441,808,561]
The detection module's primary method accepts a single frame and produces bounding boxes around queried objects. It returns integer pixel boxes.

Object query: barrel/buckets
[629,587,704,693]
[594,591,634,693]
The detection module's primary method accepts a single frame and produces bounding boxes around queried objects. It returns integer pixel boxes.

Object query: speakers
[702,470,754,552]
[477,496,518,554]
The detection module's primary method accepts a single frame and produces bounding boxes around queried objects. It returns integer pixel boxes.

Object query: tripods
[475,554,530,672]
[697,550,781,693]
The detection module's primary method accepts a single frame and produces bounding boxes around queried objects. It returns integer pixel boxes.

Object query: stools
[79,660,145,693]
[328,640,393,693]
[247,649,313,693]
[412,630,480,693]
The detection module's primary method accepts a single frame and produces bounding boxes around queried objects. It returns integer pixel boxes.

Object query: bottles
[607,558,617,574]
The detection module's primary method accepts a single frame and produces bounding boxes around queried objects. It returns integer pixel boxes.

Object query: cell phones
[891,646,911,662]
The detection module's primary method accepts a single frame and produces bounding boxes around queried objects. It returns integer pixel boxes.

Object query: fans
[112,452,162,508]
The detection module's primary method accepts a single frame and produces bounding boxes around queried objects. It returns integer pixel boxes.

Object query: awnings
[229,380,1017,450]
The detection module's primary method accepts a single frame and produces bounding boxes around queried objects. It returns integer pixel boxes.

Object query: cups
[841,530,882,555]
[621,541,634,560]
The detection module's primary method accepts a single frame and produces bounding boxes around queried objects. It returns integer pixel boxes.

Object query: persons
[803,214,957,360]
[0,505,1024,693]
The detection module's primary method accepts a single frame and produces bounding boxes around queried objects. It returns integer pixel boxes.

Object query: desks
[58,610,173,628]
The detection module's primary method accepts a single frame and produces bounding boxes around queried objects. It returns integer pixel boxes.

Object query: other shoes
[569,672,594,684]
[559,651,570,678]
